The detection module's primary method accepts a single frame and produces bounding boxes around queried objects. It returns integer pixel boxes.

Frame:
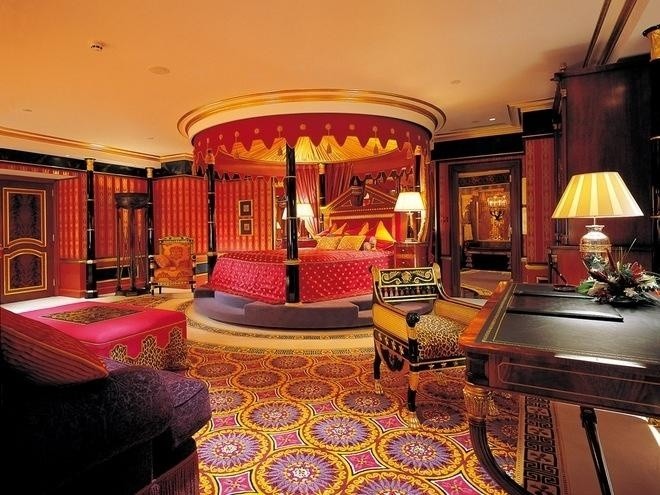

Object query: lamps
[487,197,506,221]
[393,191,424,242]
[297,203,314,239]
[549,169,645,271]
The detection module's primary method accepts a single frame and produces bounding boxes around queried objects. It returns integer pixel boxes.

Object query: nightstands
[279,237,317,249]
[394,239,429,270]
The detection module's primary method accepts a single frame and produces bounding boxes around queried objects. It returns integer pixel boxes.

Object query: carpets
[97,293,572,494]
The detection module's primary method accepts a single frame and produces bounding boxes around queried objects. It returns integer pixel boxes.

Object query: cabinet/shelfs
[547,62,660,288]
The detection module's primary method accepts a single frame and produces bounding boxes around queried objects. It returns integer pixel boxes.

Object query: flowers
[580,254,660,309]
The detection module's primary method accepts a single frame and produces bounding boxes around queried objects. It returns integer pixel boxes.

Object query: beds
[176,89,448,329]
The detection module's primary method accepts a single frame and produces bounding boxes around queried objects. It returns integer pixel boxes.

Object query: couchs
[16,300,188,374]
[0,302,216,492]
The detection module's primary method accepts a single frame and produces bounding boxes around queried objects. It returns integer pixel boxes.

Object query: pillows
[360,220,397,252]
[327,222,350,238]
[336,235,367,251]
[1,308,109,384]
[314,234,343,251]
[154,253,171,268]
[314,222,339,245]
[342,221,370,236]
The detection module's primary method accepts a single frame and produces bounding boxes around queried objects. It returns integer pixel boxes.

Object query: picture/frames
[237,219,253,236]
[238,199,254,218]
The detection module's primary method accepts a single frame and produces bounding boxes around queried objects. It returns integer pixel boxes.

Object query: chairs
[148,235,199,295]
[366,262,491,432]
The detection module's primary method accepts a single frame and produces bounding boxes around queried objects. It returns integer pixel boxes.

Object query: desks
[456,272,658,494]
